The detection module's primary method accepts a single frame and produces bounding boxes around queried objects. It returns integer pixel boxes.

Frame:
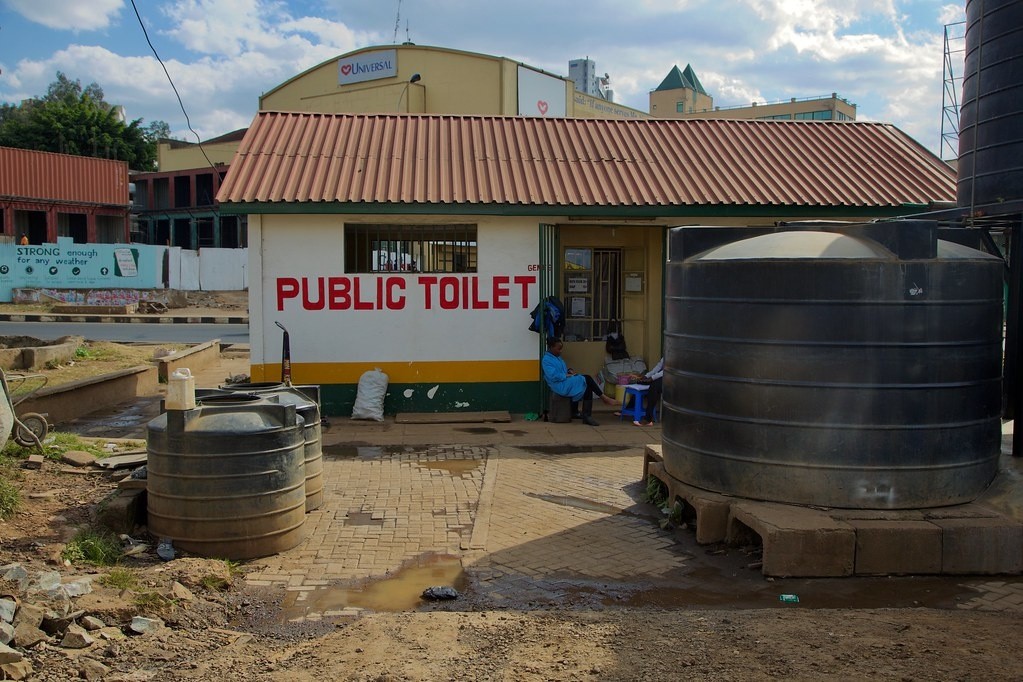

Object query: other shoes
[583,416,599,426]
[615,412,627,416]
[632,420,654,426]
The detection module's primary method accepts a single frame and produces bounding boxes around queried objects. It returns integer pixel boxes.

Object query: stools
[620,384,658,423]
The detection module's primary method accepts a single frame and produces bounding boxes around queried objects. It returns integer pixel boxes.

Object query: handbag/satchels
[606,317,626,352]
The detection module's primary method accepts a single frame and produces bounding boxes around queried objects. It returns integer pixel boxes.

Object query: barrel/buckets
[165,367,196,410]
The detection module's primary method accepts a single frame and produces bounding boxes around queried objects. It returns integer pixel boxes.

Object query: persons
[613,356,664,427]
[542,335,620,426]
